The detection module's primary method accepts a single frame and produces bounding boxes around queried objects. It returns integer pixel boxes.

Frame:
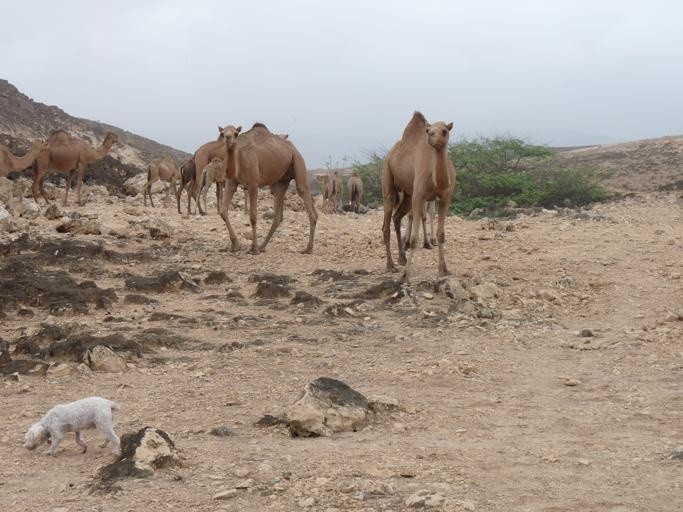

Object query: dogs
[23,396,122,458]
